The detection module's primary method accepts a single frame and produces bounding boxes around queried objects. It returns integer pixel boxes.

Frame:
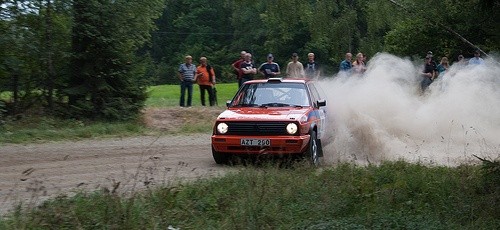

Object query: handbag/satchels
[212,87,217,100]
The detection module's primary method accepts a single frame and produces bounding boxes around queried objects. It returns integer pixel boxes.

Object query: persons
[258,53,281,79]
[286,53,304,79]
[421,51,483,91]
[196,57,216,106]
[339,53,366,73]
[302,52,320,81]
[232,50,257,88]
[178,56,197,106]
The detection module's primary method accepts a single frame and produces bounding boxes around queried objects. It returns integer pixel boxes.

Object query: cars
[210,78,335,168]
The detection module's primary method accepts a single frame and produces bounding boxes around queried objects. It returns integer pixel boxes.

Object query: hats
[293,53,297,57]
[268,54,273,58]
